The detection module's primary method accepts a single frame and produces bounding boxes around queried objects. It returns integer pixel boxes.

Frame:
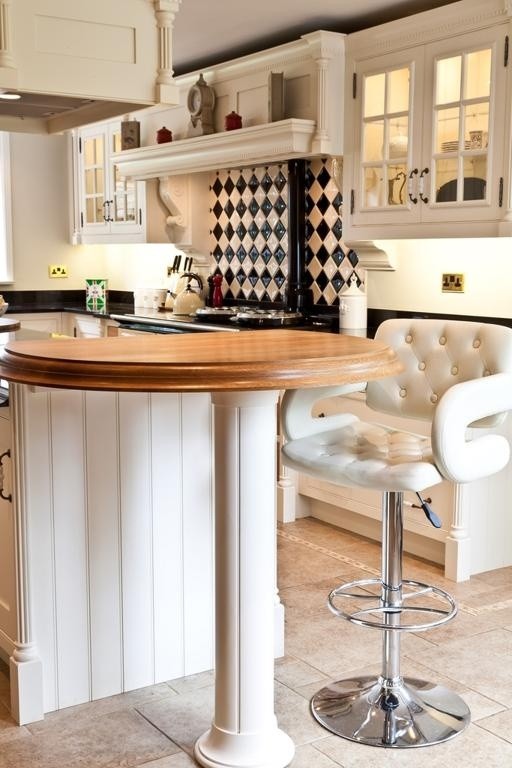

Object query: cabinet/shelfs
[15,314,115,342]
[348,21,512,244]
[67,120,196,245]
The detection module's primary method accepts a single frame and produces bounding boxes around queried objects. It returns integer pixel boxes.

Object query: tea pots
[170,273,207,316]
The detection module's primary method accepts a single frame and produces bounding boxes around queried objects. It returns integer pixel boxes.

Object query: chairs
[276,313,512,748]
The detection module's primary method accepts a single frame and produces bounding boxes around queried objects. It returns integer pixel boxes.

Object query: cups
[149,290,167,309]
[469,128,489,149]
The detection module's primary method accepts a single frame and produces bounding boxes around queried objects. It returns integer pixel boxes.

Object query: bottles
[224,111,242,131]
[207,273,224,309]
[337,273,369,330]
[155,127,172,145]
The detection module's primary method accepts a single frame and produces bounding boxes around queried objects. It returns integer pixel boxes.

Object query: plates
[441,139,471,152]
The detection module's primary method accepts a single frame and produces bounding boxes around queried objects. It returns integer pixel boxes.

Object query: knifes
[169,255,195,273]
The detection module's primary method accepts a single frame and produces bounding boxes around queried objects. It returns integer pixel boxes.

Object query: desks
[1,330,406,767]
